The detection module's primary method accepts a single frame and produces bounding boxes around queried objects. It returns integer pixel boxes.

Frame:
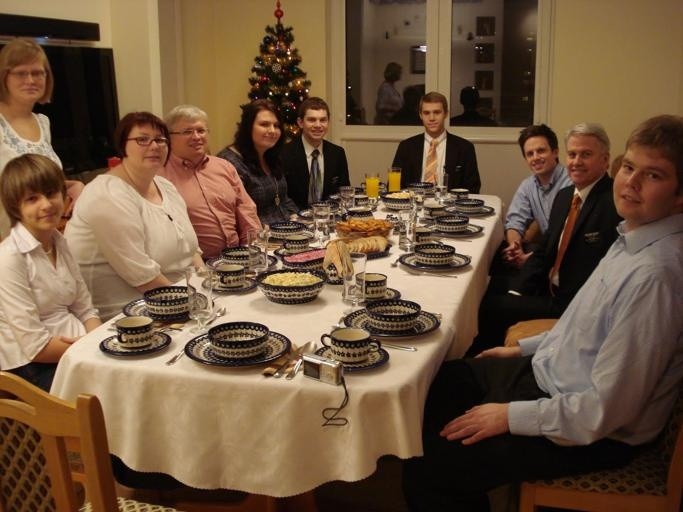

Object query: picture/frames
[410,46,426,74]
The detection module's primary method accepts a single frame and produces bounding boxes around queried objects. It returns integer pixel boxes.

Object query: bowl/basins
[143,286,194,317]
[254,269,327,304]
[363,297,422,336]
[206,322,268,359]
[412,241,458,265]
[452,197,484,215]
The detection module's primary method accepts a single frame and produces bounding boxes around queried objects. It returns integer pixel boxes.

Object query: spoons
[203,305,227,324]
[272,340,317,378]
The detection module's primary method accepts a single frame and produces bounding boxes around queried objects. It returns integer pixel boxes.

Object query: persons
[283,96,352,210]
[0,153,104,373]
[391,91,482,195]
[215,98,300,229]
[62,111,206,324]
[479,121,627,324]
[451,86,499,127]
[402,113,683,512]
[373,62,404,125]
[0,36,86,242]
[501,123,574,265]
[155,103,264,263]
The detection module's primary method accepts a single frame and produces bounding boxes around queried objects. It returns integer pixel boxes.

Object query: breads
[347,237,392,254]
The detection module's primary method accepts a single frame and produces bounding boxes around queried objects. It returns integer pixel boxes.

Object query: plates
[183,328,291,366]
[443,205,494,218]
[344,284,400,306]
[340,308,445,328]
[397,253,471,272]
[121,292,211,323]
[429,224,483,239]
[312,345,388,371]
[200,275,256,292]
[99,332,172,355]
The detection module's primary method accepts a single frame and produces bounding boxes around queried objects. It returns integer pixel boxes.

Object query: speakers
[0,13,101,43]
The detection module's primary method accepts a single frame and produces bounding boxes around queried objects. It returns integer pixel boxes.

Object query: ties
[424,140,439,185]
[549,193,582,281]
[309,148,322,203]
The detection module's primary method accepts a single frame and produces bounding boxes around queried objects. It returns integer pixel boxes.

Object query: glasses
[170,127,208,137]
[9,68,45,79]
[124,136,168,147]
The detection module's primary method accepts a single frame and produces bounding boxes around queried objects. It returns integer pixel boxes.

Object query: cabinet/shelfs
[469,13,501,122]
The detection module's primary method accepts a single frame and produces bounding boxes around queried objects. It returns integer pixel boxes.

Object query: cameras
[303,353,343,385]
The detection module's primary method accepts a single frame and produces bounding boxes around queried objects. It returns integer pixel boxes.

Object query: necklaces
[45,237,55,255]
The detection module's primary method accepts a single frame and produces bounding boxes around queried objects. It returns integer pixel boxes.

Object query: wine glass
[181,267,220,332]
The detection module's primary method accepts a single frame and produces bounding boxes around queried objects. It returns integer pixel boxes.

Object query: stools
[505,318,557,346]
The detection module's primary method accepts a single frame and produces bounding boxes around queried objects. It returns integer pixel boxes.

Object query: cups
[320,328,383,365]
[214,264,244,288]
[347,269,388,300]
[113,313,153,352]
[202,166,468,273]
[341,251,367,305]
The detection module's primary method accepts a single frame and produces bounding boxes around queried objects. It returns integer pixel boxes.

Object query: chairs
[517,420,682,512]
[0,371,176,511]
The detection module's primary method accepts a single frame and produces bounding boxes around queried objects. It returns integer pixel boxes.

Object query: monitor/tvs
[0,43,122,176]
